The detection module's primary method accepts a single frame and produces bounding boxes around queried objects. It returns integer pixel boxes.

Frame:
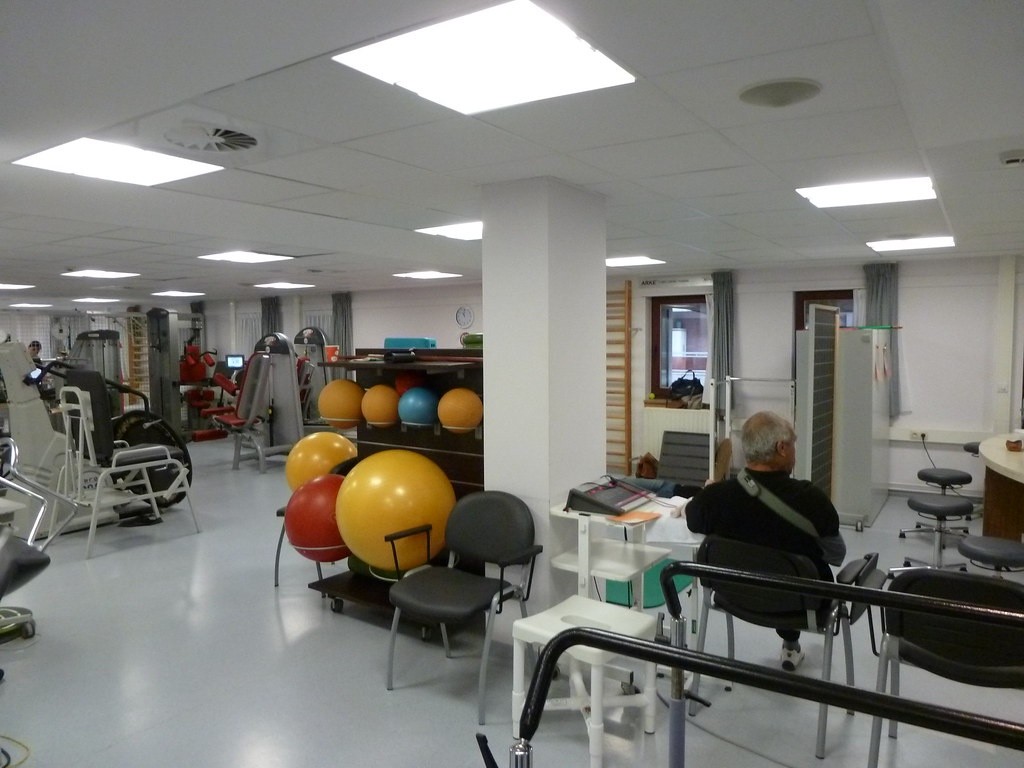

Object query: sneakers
[780,642,804,672]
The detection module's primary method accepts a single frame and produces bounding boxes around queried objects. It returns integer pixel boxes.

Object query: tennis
[649,393,655,400]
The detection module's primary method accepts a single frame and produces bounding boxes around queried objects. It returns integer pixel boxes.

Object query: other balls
[284,366,483,571]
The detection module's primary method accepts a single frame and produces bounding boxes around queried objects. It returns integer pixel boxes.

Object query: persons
[684,407,848,672]
[28,339,42,364]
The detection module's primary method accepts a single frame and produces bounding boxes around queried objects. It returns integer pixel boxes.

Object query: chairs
[45,368,201,558]
[868,570,1023,768]
[383,489,543,726]
[274,456,363,599]
[178,343,316,475]
[689,534,881,759]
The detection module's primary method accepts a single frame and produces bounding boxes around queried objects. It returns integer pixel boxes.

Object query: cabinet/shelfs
[550,503,671,696]
[832,328,889,528]
[310,347,484,640]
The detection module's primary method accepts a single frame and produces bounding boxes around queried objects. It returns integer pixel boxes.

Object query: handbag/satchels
[666,369,704,409]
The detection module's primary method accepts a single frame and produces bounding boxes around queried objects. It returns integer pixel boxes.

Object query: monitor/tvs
[225,354,245,369]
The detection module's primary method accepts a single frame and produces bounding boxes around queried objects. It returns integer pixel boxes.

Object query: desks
[978,429,1024,544]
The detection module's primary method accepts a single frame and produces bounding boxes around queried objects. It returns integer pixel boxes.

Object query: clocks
[455,305,474,328]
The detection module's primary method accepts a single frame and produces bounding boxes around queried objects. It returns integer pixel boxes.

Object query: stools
[885,492,974,580]
[956,535,1024,579]
[962,441,984,520]
[513,593,657,768]
[899,468,972,550]
